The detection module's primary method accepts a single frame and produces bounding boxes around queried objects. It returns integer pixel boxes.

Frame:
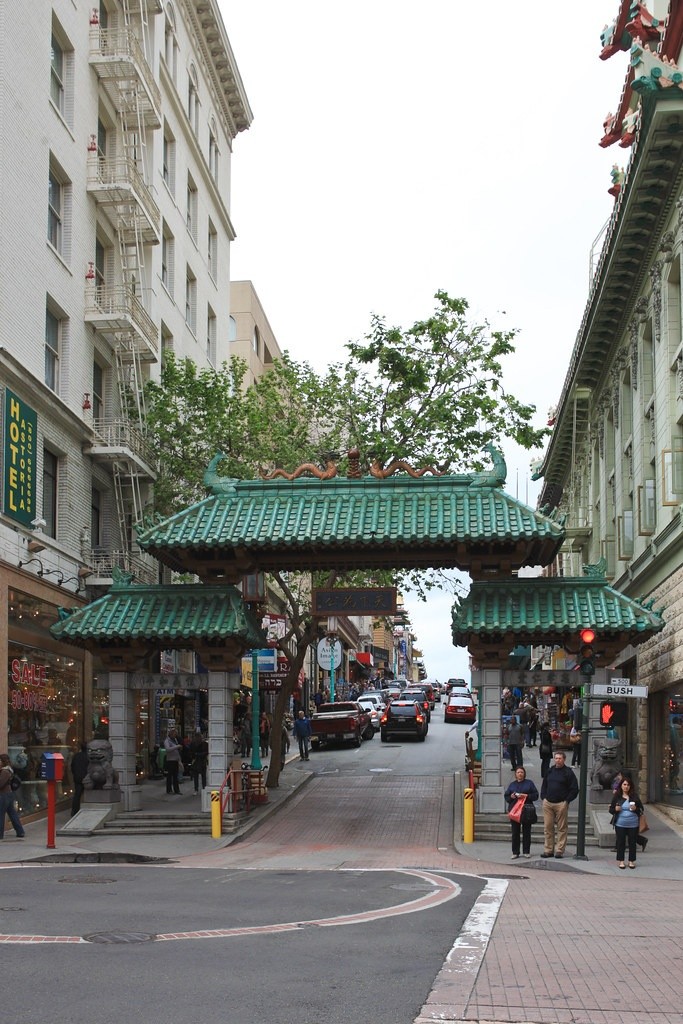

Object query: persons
[236,713,291,759]
[504,767,539,859]
[609,769,649,869]
[293,698,312,761]
[0,754,25,839]
[570,721,582,768]
[152,729,207,796]
[500,686,587,760]
[539,722,553,779]
[540,753,579,859]
[308,674,395,717]
[504,716,525,771]
[71,742,90,817]
[234,694,252,732]
[467,720,478,748]
[20,721,77,811]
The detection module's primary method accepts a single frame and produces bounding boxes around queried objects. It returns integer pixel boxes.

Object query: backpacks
[5,768,21,790]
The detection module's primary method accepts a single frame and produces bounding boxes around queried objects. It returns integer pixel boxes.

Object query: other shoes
[17,832,24,838]
[175,791,182,795]
[642,837,648,852]
[300,757,310,761]
[610,848,618,852]
[552,851,565,858]
[509,854,519,860]
[241,754,250,758]
[192,791,199,796]
[540,852,555,858]
[618,861,627,870]
[262,752,268,758]
[627,861,635,869]
[167,790,176,795]
[523,853,531,859]
[510,767,516,772]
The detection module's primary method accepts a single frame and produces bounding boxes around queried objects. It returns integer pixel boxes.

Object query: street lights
[324,617,339,702]
[398,655,404,674]
[241,570,268,770]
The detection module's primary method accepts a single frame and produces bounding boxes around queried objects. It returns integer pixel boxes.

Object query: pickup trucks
[309,701,374,748]
[444,678,468,694]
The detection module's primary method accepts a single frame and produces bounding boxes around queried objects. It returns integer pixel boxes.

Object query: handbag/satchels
[639,809,650,834]
[519,799,537,825]
[539,740,552,756]
[508,795,527,823]
[570,735,582,744]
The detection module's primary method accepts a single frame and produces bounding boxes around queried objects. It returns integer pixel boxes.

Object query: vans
[421,679,441,702]
[407,683,437,711]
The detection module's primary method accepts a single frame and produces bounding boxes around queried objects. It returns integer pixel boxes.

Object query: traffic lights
[578,629,596,675]
[600,701,629,726]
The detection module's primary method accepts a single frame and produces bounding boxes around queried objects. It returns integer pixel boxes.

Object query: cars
[443,687,478,724]
[358,702,381,732]
[369,680,410,705]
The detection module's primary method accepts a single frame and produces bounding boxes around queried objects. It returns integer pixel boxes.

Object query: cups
[629,802,635,811]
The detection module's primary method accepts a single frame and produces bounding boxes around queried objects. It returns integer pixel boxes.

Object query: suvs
[380,700,428,742]
[357,693,387,719]
[397,689,431,723]
